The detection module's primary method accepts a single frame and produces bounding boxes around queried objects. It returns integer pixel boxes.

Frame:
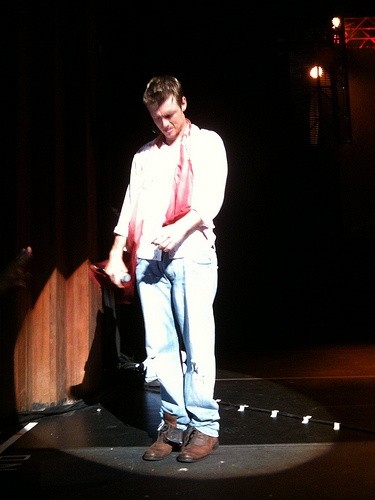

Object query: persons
[102,75,229,465]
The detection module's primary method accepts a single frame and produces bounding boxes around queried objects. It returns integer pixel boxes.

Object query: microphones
[89,265,131,283]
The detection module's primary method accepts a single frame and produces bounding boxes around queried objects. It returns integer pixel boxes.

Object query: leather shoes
[177,430,219,462]
[141,411,184,460]
